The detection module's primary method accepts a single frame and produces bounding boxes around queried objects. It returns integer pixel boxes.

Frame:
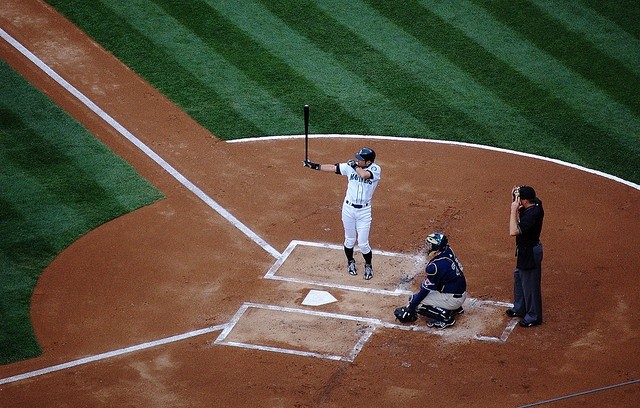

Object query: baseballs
[304,105,310,161]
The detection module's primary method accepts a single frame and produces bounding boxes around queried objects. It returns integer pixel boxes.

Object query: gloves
[302,160,319,170]
[346,160,359,170]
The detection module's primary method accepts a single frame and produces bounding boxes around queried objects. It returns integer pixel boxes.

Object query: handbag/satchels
[516,246,538,271]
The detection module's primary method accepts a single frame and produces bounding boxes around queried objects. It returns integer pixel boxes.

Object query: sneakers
[348,260,357,275]
[506,309,520,317]
[448,306,464,317]
[426,317,455,328]
[519,318,542,327]
[364,263,373,280]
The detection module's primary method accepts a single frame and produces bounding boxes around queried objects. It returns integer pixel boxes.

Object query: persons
[401,233,467,329]
[302,147,382,280]
[506,185,544,328]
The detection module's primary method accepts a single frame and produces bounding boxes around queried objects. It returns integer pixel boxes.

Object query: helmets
[425,233,448,260]
[355,148,375,161]
[513,185,540,209]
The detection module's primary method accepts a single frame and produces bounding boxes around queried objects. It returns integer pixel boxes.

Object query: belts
[346,201,368,208]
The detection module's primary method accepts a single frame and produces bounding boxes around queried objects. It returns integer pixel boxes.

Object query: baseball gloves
[394,306,418,323]
